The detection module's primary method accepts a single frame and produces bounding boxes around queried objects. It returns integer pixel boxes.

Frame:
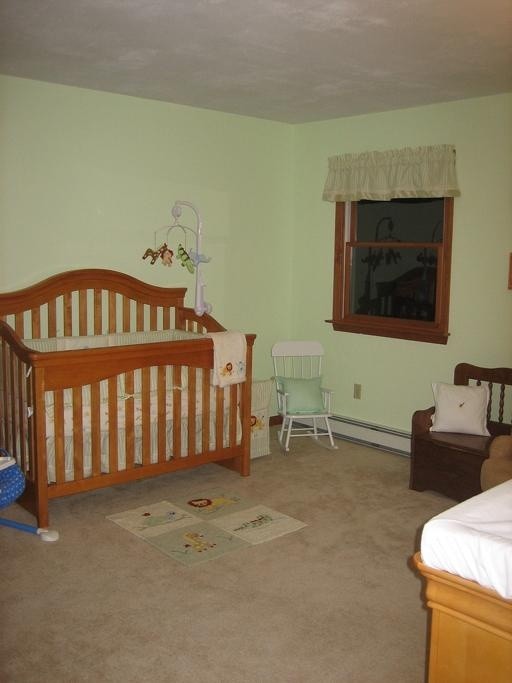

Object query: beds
[412,479,512,683]
[0,268,257,529]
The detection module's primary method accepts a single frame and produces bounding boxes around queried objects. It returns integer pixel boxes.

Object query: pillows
[429,381,491,437]
[276,374,326,415]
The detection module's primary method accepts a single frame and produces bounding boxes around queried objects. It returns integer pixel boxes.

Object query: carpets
[106,486,309,568]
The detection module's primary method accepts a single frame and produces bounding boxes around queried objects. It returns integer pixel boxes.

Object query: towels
[206,332,247,388]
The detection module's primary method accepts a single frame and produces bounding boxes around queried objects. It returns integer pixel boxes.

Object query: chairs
[409,363,512,502]
[271,340,338,452]
